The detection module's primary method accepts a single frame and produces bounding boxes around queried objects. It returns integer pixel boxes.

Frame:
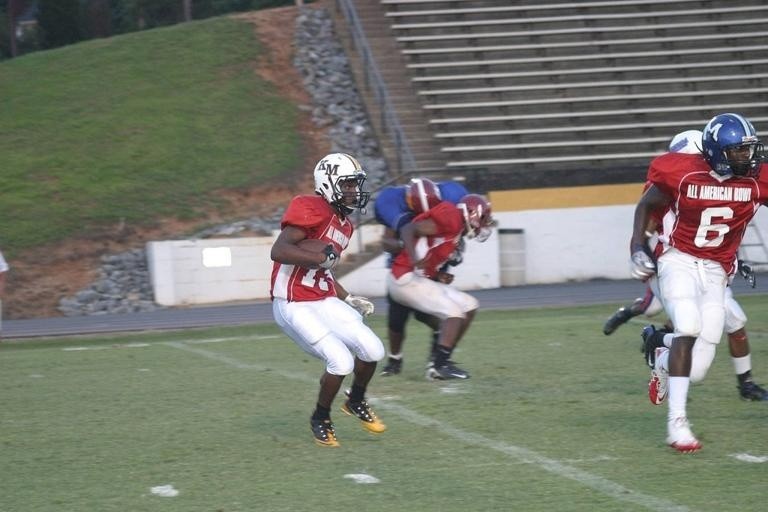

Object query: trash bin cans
[499,228,526,287]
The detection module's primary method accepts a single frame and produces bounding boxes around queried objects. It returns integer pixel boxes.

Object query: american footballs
[296,239,338,269]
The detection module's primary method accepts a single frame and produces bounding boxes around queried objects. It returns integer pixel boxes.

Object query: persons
[600,110,767,454]
[373,175,467,375]
[268,151,388,448]
[376,192,493,380]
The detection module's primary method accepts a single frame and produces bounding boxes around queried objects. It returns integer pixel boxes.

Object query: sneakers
[309,415,338,447]
[648,347,669,406]
[643,326,664,369]
[422,359,470,381]
[665,420,700,452]
[382,354,401,376]
[605,305,632,334]
[737,383,768,404]
[341,398,387,434]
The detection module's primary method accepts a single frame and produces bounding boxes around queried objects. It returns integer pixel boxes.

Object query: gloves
[319,244,340,270]
[346,294,373,318]
[629,252,656,282]
[740,261,755,289]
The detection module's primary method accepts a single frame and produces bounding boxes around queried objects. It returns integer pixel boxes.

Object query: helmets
[702,113,766,182]
[312,153,371,216]
[458,194,496,242]
[405,177,441,212]
[670,130,704,153]
